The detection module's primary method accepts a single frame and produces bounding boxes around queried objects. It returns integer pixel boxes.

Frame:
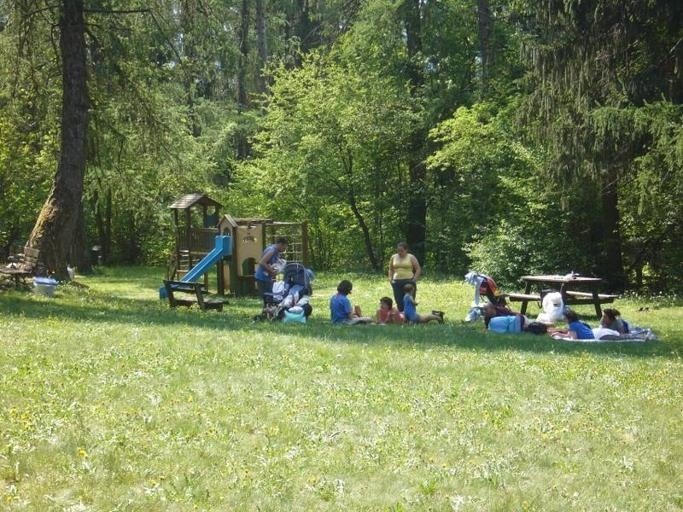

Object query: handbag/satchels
[284,309,307,325]
[487,316,522,334]
[529,321,547,335]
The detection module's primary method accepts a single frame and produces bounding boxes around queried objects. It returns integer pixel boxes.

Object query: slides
[159,248,224,298]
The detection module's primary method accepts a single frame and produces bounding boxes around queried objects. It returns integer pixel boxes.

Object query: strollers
[254,263,314,323]
[468,271,525,329]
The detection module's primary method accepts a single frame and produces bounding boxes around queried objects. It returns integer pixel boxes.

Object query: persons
[266,258,286,277]
[386,241,421,313]
[401,281,420,326]
[479,290,653,344]
[253,236,289,319]
[262,302,313,324]
[375,296,445,326]
[329,280,372,327]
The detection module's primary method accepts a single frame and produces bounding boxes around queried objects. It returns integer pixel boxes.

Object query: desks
[520,275,603,322]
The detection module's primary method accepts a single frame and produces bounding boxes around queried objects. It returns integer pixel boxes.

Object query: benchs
[162,280,226,313]
[565,290,619,321]
[0,247,41,289]
[508,293,542,316]
